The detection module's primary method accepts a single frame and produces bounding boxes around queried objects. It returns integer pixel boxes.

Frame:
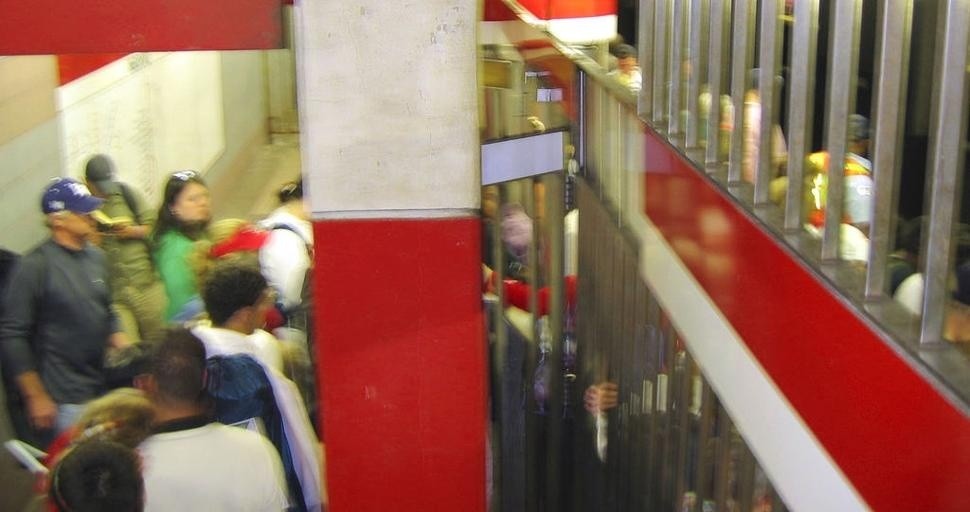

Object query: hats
[615,43,638,59]
[844,113,869,142]
[42,178,107,214]
[85,154,123,195]
[746,66,760,88]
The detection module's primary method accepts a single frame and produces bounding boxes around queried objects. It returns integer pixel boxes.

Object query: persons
[0,151,328,512]
[468,33,970,511]
